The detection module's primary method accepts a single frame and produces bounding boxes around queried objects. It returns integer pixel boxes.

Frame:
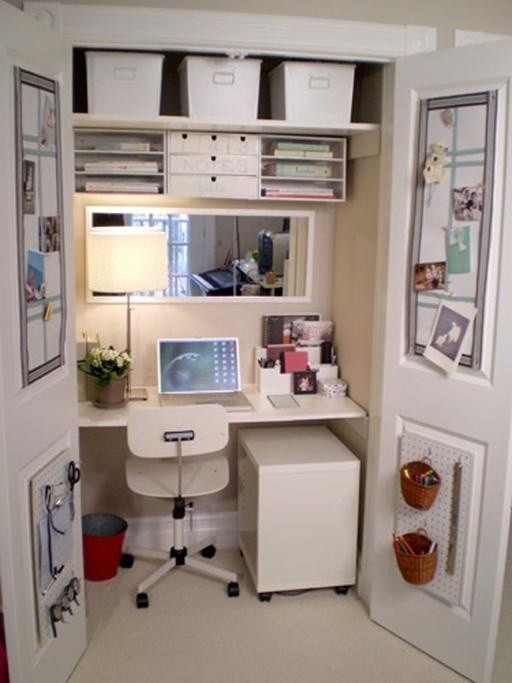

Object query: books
[84,161,158,172]
[264,162,332,178]
[269,148,333,157]
[95,142,150,151]
[270,140,330,150]
[270,194,336,198]
[262,187,334,195]
[268,393,300,407]
[85,177,160,193]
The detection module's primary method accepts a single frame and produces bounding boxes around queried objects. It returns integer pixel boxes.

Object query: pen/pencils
[258,358,278,368]
[391,532,437,555]
[404,467,440,487]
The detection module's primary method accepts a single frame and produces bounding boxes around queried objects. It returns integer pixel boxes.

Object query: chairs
[124,402,243,610]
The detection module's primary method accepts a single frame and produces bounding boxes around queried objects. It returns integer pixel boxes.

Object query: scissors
[68,461,80,521]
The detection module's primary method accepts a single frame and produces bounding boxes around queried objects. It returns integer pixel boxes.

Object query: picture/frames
[293,369,317,394]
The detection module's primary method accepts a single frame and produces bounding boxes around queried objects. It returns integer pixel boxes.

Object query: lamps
[85,228,169,400]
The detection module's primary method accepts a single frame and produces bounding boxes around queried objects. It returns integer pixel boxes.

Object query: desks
[232,256,285,296]
[76,380,369,429]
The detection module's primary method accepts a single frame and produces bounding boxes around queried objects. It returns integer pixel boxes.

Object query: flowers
[76,343,134,388]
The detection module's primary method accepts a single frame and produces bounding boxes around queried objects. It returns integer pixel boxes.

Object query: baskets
[393,527,437,584]
[399,457,441,510]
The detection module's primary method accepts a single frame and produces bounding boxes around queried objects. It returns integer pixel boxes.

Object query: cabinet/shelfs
[234,425,362,600]
[73,127,347,203]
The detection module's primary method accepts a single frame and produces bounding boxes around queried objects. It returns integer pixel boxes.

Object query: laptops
[157,337,253,413]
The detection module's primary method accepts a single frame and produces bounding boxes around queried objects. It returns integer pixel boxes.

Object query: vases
[91,377,128,407]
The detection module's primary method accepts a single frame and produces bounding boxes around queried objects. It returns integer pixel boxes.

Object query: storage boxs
[269,62,358,120]
[177,54,263,118]
[81,50,166,117]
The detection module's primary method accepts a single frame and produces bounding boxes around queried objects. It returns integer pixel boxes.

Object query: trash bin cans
[81,513,129,582]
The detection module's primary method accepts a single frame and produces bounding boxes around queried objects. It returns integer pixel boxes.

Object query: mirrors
[81,206,315,307]
